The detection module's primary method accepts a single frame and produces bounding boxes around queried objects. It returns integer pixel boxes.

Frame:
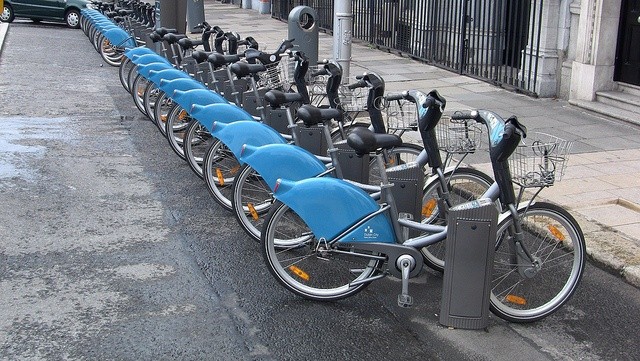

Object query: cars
[0,0,91,28]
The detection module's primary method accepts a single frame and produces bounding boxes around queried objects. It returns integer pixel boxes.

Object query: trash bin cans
[287,5,319,85]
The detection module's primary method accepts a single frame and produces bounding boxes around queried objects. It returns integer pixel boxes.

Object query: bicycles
[231,91,504,270]
[261,112,587,323]
